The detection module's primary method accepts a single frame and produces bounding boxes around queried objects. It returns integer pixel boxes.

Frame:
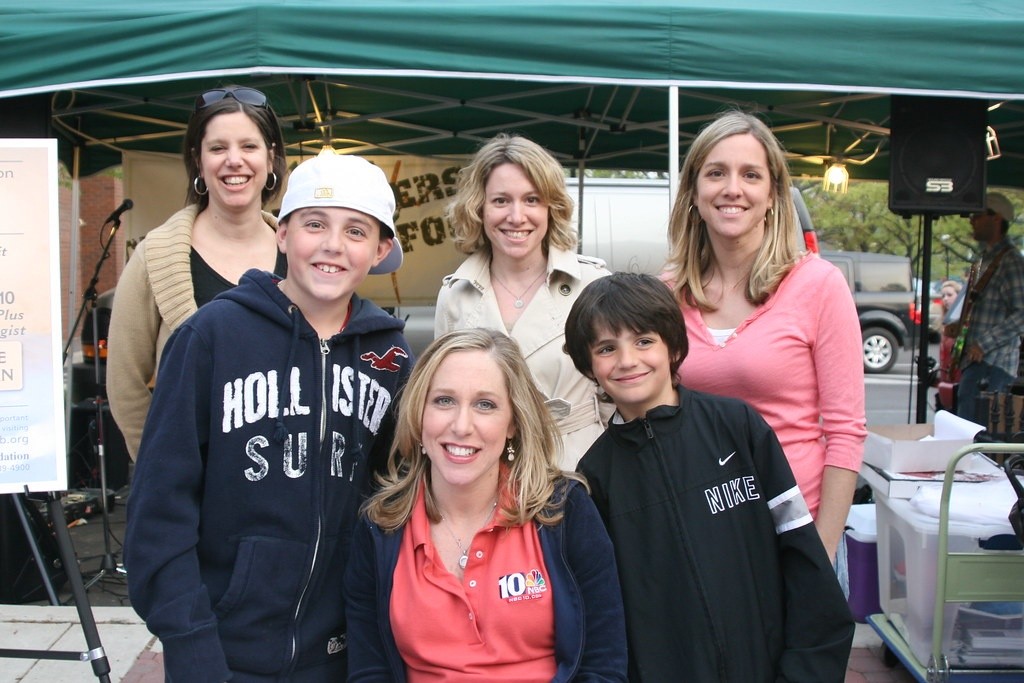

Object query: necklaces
[491,267,547,308]
[434,495,499,568]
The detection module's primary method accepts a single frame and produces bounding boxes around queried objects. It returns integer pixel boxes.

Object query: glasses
[191,88,269,110]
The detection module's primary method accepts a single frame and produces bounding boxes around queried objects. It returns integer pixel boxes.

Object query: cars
[819,248,921,375]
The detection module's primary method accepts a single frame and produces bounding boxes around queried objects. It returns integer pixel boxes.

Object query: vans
[564,177,818,273]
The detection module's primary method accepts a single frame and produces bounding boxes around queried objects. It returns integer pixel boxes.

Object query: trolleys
[865,442,1024,683]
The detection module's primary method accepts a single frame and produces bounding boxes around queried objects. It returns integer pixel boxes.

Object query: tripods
[59,220,127,606]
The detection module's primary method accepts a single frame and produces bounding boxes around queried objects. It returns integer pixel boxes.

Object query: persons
[943,193,1024,428]
[565,272,856,683]
[656,102,866,599]
[107,82,287,467]
[432,131,620,477]
[360,326,631,683]
[122,152,413,683]
[939,279,962,311]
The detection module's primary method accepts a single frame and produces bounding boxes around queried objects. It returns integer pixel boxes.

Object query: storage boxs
[844,423,1024,668]
[27,487,115,525]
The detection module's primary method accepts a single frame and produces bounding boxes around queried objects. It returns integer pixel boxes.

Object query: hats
[277,155,403,274]
[985,191,1014,221]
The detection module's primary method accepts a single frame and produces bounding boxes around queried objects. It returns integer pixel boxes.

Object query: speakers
[69,396,130,487]
[890,94,987,218]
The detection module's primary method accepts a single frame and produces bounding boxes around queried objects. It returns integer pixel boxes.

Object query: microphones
[104,198,133,223]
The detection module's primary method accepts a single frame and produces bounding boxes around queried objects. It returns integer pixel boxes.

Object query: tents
[0,0,1024,498]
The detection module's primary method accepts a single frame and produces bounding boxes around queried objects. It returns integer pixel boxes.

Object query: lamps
[821,156,849,195]
[316,135,338,158]
